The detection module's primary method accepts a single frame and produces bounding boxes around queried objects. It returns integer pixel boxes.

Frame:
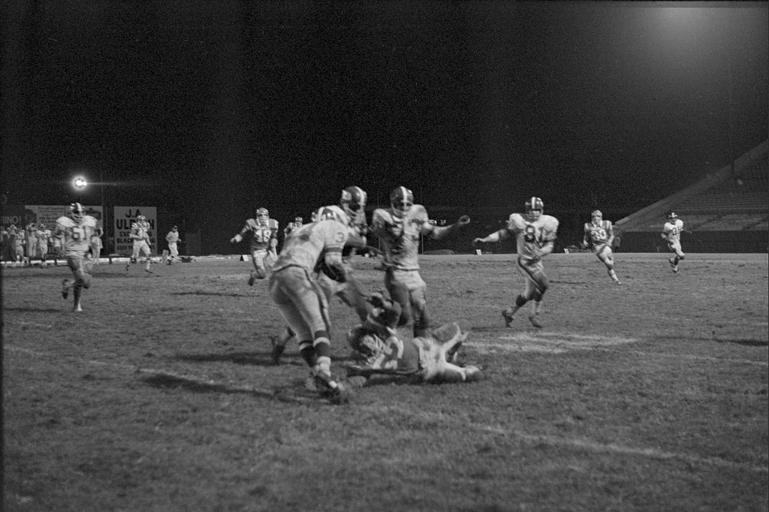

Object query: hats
[173,226,177,228]
[591,210,603,224]
[524,196,544,222]
[666,212,678,223]
[346,325,385,359]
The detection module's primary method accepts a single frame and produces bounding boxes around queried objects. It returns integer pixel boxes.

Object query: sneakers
[74,305,83,312]
[63,280,68,299]
[668,258,674,265]
[271,335,285,364]
[615,280,621,285]
[502,309,543,328]
[126,263,130,272]
[306,369,343,404]
[673,269,677,274]
[145,268,152,273]
[248,274,254,286]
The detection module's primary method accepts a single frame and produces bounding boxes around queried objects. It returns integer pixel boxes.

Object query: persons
[360,185,473,340]
[283,217,303,245]
[165,225,181,264]
[269,184,377,365]
[228,208,279,287]
[331,292,483,393]
[0,221,105,269]
[267,205,371,406]
[472,195,558,331]
[579,209,620,283]
[49,201,98,314]
[659,211,685,272]
[123,214,154,274]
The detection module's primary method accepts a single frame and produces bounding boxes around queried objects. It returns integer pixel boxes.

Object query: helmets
[70,202,85,222]
[295,217,302,225]
[257,207,269,226]
[389,186,414,217]
[137,215,146,226]
[311,185,368,226]
